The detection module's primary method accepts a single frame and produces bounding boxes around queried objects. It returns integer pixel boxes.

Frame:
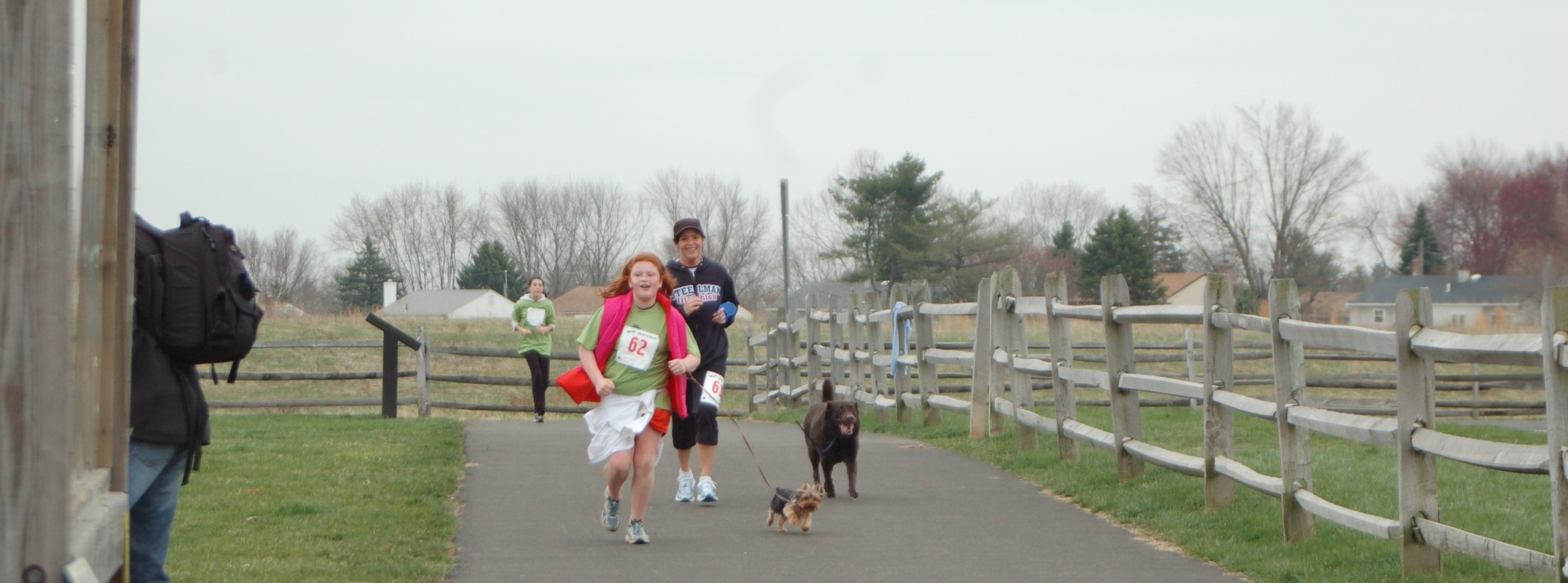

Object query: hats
[673,218,704,237]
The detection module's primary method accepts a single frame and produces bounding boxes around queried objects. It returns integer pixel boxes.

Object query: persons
[511,277,557,422]
[125,213,210,583]
[666,218,740,501]
[577,253,701,544]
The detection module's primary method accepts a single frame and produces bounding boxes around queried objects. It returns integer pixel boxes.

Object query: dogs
[767,481,824,531]
[800,373,861,497]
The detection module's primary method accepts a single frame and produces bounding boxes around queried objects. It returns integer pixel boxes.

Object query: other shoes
[534,413,544,423]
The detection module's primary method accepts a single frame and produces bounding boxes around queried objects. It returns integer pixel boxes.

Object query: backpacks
[134,209,265,365]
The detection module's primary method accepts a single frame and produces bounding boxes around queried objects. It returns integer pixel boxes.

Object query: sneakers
[625,518,650,544]
[601,486,622,531]
[697,480,718,502]
[675,475,695,502]
